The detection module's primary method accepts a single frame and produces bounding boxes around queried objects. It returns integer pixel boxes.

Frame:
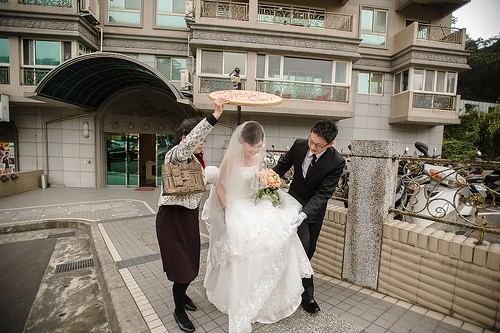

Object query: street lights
[228,66,242,126]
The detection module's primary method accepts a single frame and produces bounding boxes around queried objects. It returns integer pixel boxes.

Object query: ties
[304,154,317,180]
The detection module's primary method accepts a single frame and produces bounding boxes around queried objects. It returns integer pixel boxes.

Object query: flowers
[255,168,282,208]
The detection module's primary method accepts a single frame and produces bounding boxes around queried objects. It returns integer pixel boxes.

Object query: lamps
[83,121,90,138]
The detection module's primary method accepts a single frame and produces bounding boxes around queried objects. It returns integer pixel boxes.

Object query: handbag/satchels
[160,147,207,197]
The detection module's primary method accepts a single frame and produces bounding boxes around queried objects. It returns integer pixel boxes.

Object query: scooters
[262,143,294,183]
[335,140,500,237]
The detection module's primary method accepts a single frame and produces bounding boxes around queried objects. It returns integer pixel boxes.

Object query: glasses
[307,135,332,149]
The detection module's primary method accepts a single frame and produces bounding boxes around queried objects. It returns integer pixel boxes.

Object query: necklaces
[241,157,251,165]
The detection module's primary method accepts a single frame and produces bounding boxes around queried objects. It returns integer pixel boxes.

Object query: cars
[107,139,138,164]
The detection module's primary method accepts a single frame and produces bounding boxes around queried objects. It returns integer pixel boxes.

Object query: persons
[156,97,229,333]
[3,151,9,169]
[272,119,345,314]
[202,121,314,333]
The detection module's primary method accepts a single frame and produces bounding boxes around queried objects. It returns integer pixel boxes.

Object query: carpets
[135,188,155,191]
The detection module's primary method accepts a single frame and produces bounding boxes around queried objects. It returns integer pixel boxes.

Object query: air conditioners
[180,68,192,90]
[184,1,194,16]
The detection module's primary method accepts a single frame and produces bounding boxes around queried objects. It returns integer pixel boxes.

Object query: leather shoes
[184,295,196,311]
[302,296,320,314]
[173,310,195,333]
